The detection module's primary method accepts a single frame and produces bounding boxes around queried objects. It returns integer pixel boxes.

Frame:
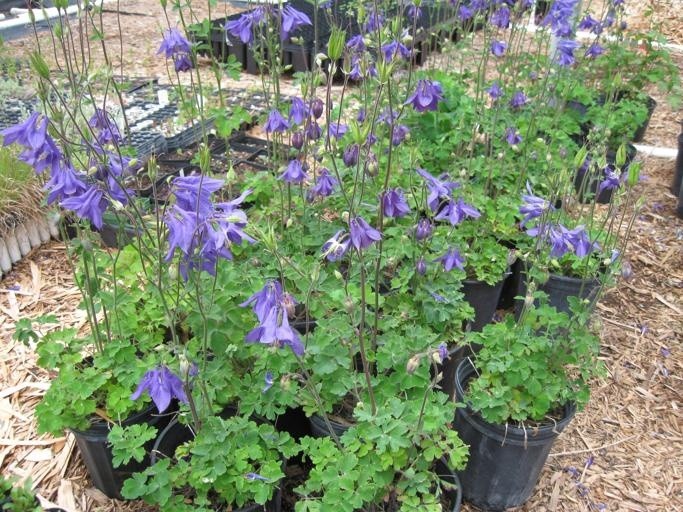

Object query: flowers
[0,0,682,512]
